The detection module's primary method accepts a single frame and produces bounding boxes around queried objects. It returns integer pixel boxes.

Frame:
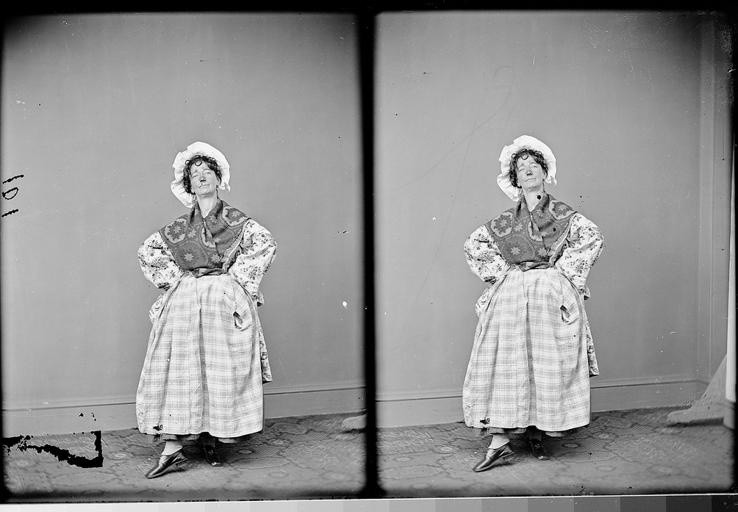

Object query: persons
[137,140,279,478]
[461,134,606,471]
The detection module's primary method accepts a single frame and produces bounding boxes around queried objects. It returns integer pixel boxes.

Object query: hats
[497,134,557,203]
[170,142,231,208]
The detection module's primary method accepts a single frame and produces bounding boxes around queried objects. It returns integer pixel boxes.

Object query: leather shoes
[146,444,222,479]
[474,437,549,473]
